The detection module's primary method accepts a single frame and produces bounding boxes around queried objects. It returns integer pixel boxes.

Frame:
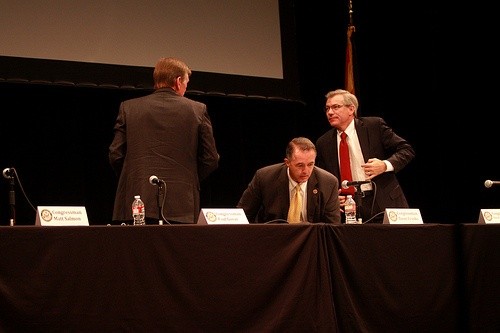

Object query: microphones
[341,180,371,189]
[3,167,15,179]
[149,175,164,186]
[483,180,500,188]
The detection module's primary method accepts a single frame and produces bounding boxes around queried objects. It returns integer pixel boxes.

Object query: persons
[314,90,416,223]
[238,138,341,223]
[109,57,220,224]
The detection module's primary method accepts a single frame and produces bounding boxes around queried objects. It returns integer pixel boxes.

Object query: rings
[368,172,370,175]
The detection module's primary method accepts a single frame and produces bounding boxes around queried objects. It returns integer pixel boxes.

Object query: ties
[287,184,302,223]
[339,132,354,199]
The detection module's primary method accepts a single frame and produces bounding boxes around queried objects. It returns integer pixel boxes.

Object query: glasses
[325,105,350,110]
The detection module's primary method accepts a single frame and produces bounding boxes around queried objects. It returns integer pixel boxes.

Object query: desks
[0,223,500,333]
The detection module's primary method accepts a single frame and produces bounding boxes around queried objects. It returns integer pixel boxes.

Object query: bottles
[132,195,145,225]
[344,195,357,224]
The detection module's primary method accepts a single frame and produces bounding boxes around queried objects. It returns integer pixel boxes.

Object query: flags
[344,28,360,98]
